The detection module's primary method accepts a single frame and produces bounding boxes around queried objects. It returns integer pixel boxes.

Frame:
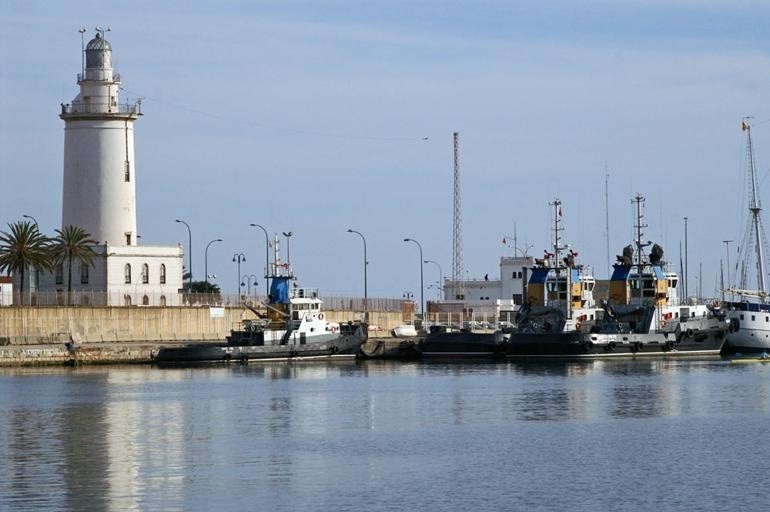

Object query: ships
[412,197,606,359]
[501,191,730,362]
[723,114,770,359]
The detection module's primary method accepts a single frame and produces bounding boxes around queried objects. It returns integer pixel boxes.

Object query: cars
[367,319,517,337]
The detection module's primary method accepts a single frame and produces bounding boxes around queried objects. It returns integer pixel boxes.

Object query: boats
[149,232,369,364]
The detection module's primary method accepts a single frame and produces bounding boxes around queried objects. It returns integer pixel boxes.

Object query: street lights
[404,238,424,321]
[283,230,294,289]
[403,292,414,300]
[176,219,193,307]
[240,275,258,306]
[424,258,442,302]
[508,243,535,264]
[347,229,368,299]
[79,28,87,81]
[250,223,270,306]
[95,26,113,81]
[231,253,246,307]
[203,238,222,305]
[24,214,40,307]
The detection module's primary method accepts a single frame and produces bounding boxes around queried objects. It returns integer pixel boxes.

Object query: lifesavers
[576,323,580,329]
[319,314,323,319]
[661,320,666,328]
[331,327,336,333]
